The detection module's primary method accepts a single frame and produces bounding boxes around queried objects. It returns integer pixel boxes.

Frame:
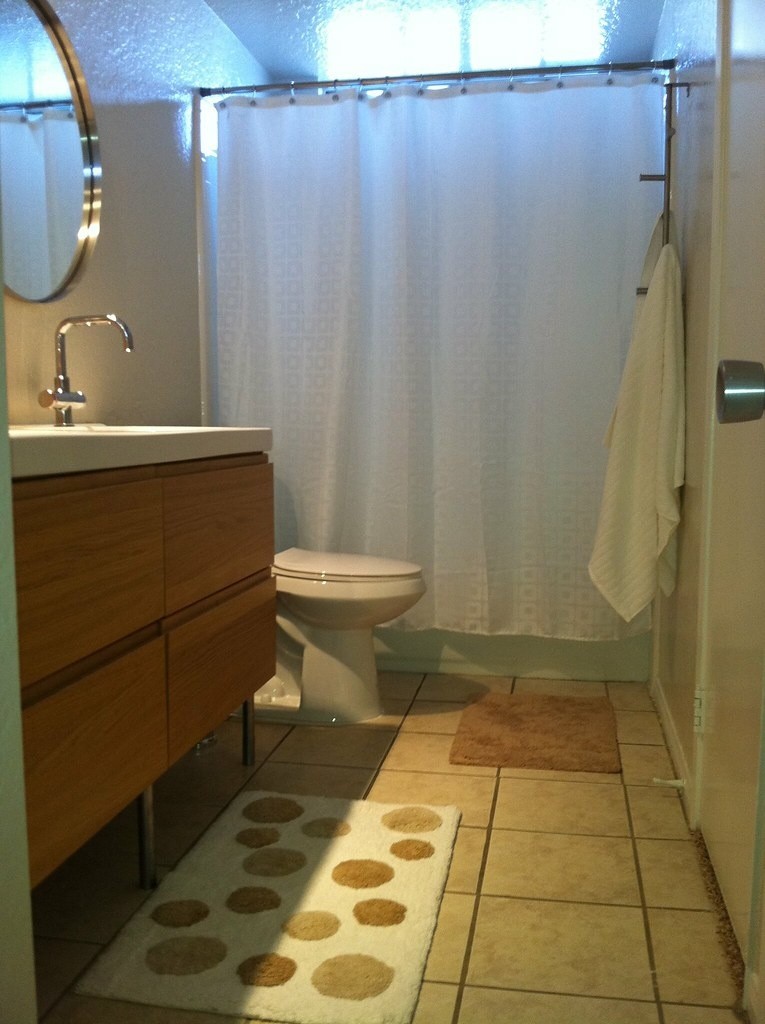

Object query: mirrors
[0,0,103,304]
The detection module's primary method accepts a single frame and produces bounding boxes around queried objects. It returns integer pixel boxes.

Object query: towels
[586,243,686,625]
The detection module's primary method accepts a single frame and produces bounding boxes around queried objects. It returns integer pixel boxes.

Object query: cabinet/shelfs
[12,453,278,905]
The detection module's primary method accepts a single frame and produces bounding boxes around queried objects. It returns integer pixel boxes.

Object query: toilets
[273,545,429,726]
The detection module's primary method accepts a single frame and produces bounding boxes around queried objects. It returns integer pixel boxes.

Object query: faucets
[38,315,135,426]
